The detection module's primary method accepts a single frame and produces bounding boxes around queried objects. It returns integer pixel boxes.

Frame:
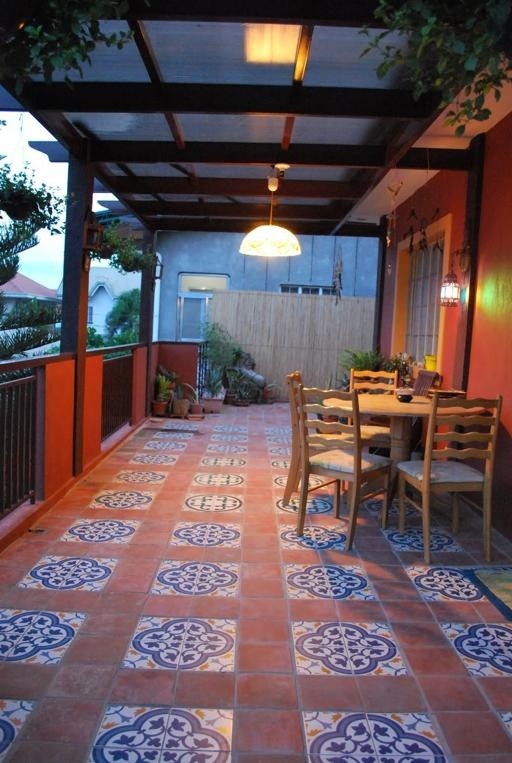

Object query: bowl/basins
[397,395,412,402]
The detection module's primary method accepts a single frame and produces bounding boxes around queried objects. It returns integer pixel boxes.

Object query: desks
[319,393,487,533]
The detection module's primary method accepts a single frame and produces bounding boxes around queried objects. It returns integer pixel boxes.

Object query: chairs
[295,383,393,550]
[397,392,502,565]
[348,368,468,506]
[283,369,356,521]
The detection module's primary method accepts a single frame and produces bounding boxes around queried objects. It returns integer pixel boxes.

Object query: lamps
[239,164,302,258]
[439,241,471,306]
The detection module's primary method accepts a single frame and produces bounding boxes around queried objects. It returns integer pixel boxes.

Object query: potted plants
[151,373,172,416]
[198,321,239,413]
